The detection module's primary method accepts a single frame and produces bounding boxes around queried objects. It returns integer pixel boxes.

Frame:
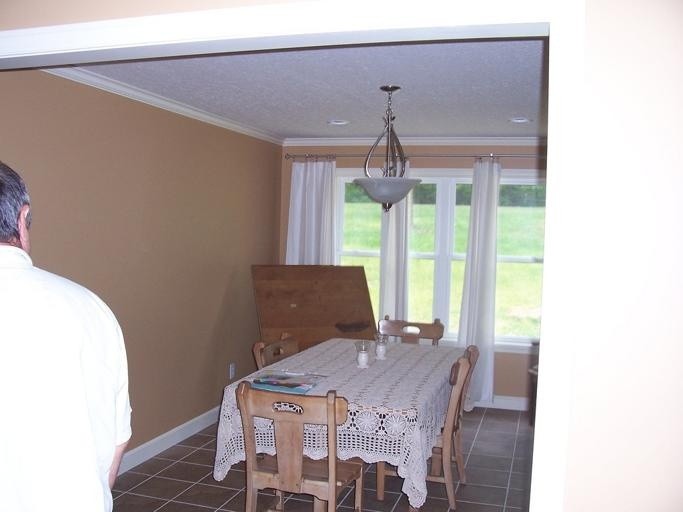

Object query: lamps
[354,86,422,212]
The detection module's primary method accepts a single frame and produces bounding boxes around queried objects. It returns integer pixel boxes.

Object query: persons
[0,156,132,510]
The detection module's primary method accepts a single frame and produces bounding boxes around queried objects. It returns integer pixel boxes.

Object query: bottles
[355,334,389,368]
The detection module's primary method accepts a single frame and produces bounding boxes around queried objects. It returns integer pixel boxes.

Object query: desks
[215,337,480,508]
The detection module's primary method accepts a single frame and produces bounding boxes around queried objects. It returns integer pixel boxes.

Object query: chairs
[251,327,299,368]
[229,378,365,509]
[375,342,479,511]
[376,316,444,345]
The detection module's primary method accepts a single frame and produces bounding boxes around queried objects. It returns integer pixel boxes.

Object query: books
[248,367,327,396]
